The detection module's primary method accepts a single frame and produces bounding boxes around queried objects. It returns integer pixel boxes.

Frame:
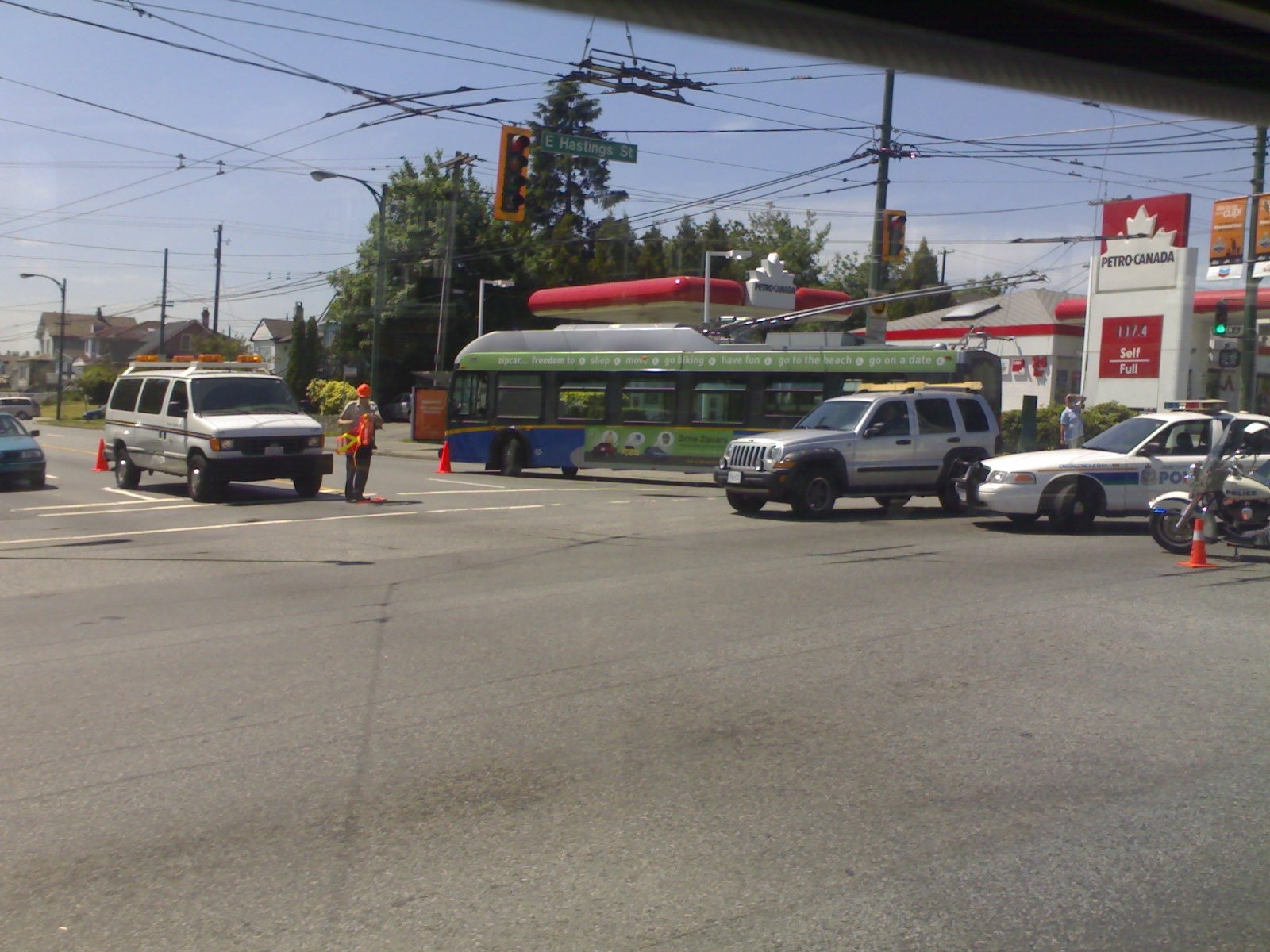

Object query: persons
[1060,394,1087,449]
[338,384,383,503]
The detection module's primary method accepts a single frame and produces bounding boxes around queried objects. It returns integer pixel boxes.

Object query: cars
[0,396,41,420]
[966,398,1270,534]
[382,393,412,422]
[0,413,45,489]
[82,404,107,420]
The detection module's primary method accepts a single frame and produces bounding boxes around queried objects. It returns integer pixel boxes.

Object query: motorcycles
[1147,417,1270,554]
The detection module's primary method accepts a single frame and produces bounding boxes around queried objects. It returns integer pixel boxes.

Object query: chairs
[1195,430,1210,455]
[1167,432,1194,455]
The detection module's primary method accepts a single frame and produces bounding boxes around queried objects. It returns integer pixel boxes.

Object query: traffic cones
[436,441,457,474]
[1177,520,1219,568]
[91,438,110,471]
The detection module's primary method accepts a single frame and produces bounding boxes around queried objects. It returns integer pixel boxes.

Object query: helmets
[356,384,371,398]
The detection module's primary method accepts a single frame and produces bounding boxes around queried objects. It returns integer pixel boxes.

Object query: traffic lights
[882,209,907,262]
[493,124,531,222]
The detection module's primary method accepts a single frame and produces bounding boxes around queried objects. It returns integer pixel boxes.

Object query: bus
[438,325,1002,481]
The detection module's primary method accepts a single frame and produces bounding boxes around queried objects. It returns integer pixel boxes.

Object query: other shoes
[356,495,370,501]
[346,496,356,502]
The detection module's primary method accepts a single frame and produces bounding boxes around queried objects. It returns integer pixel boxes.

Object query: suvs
[105,353,334,502]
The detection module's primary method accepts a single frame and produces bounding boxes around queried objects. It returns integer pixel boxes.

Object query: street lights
[705,249,753,324]
[478,278,515,339]
[938,247,954,284]
[20,273,65,420]
[311,171,387,431]
